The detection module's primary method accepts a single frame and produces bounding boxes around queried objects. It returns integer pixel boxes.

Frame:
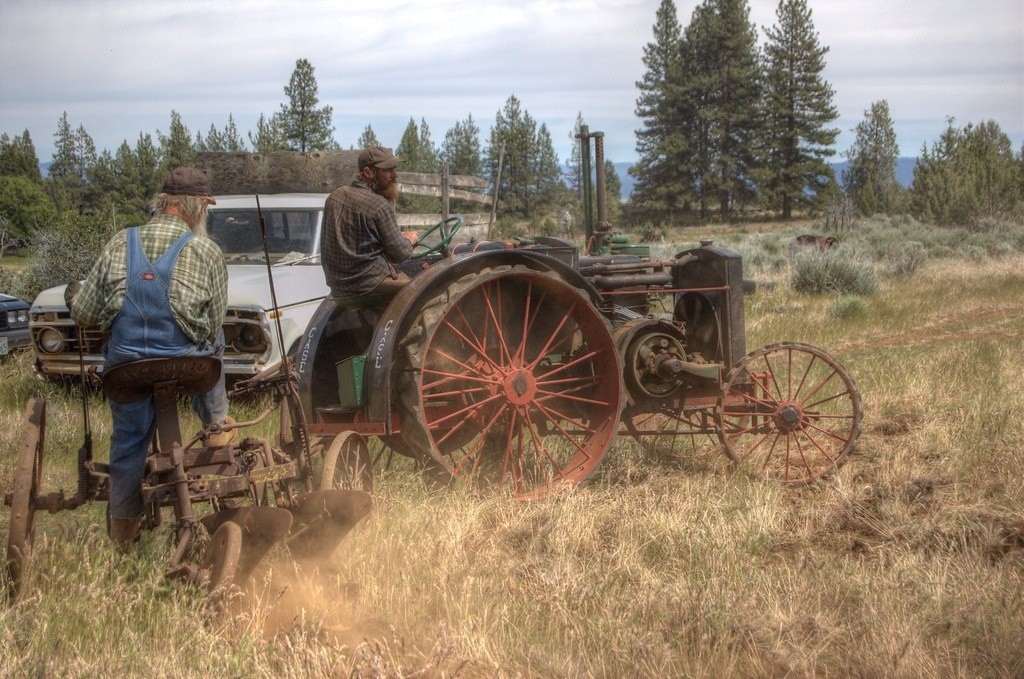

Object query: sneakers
[203,415,238,447]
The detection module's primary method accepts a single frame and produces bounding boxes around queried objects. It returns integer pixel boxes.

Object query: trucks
[29,152,500,383]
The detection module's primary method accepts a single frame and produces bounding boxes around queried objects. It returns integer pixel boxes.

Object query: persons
[63,166,237,583]
[319,146,422,315]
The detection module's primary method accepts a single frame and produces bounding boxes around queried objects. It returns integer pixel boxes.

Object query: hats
[357,146,402,170]
[161,165,216,205]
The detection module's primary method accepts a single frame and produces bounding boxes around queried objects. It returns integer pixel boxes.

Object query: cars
[1,294,33,365]
